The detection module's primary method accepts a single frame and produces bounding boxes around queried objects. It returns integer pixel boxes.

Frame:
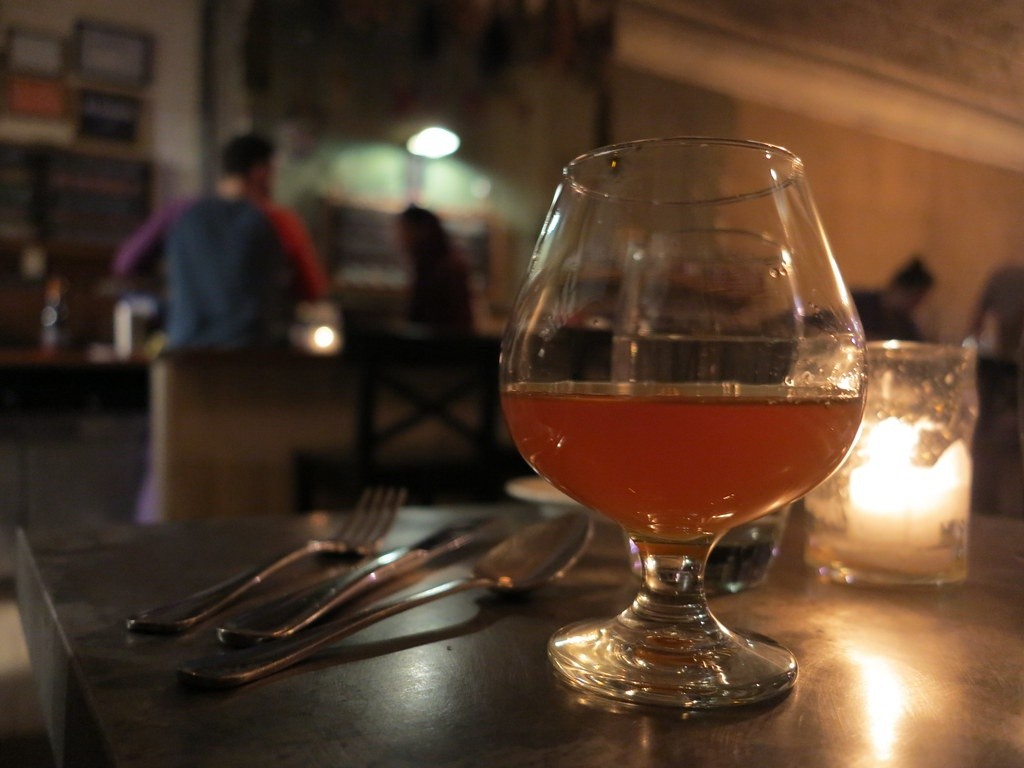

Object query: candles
[842,415,971,556]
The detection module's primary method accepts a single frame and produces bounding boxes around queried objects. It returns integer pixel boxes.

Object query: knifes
[216,518,501,643]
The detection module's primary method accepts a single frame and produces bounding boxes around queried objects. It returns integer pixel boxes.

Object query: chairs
[285,301,510,518]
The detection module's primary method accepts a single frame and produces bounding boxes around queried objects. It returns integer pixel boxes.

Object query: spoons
[171,512,595,691]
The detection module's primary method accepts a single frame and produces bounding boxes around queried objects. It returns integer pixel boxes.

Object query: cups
[806,340,975,592]
[609,226,804,593]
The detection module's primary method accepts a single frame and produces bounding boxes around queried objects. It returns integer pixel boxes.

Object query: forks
[127,489,407,632]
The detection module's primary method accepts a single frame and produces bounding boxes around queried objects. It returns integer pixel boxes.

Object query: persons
[112,133,323,528]
[960,264,1024,522]
[392,201,473,339]
[845,254,935,344]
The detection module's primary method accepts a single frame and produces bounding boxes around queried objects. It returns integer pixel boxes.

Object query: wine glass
[499,135,868,716]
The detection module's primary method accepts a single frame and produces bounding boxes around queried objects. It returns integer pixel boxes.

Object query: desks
[10,501,1023,768]
